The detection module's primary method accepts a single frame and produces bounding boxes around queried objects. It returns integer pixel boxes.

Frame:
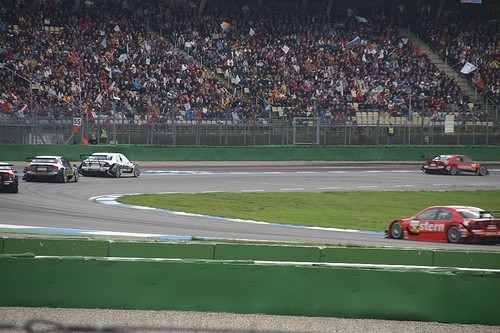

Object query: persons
[0,0,500,126]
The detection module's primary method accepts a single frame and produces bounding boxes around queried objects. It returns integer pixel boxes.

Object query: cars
[420,154,489,176]
[22,155,80,183]
[77,152,141,178]
[384,205,500,243]
[0,162,19,193]
